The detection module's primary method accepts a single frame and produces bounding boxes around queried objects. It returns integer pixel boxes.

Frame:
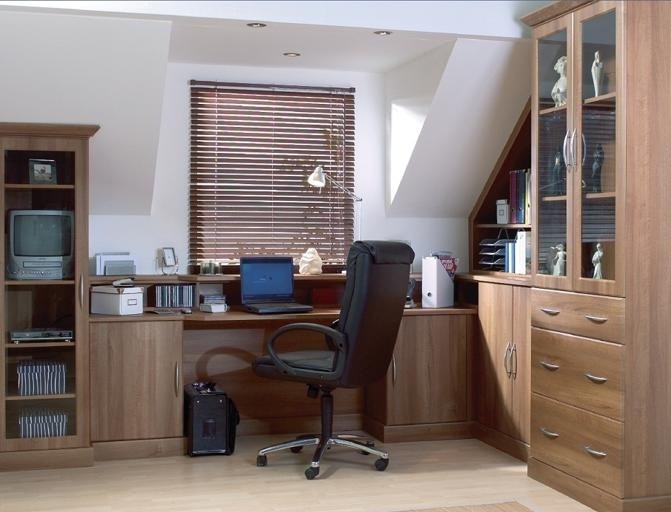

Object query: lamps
[306,164,363,205]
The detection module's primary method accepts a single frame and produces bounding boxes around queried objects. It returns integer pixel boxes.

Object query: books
[505,231,531,274]
[200,304,227,313]
[155,285,194,308]
[509,169,532,224]
[204,295,228,304]
[111,277,136,288]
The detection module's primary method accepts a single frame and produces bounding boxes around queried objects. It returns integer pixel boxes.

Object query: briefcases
[183,379,239,457]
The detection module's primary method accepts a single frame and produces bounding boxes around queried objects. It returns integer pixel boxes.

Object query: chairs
[252,239,415,481]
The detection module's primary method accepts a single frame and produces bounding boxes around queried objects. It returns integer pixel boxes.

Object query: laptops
[240,256,313,314]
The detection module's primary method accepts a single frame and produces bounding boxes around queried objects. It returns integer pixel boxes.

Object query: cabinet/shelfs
[472,104,531,277]
[532,0,627,298]
[531,287,627,497]
[476,281,531,449]
[1,144,79,449]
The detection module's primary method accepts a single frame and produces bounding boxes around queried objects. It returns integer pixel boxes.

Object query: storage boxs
[92,285,146,317]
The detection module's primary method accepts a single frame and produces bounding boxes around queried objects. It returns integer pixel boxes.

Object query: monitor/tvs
[6,209,75,279]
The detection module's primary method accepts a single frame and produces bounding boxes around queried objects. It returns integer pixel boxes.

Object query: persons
[550,56,567,107]
[552,144,564,196]
[553,244,567,276]
[590,143,604,192]
[592,243,604,279]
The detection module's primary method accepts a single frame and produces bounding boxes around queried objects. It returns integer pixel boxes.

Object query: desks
[91,273,475,461]
